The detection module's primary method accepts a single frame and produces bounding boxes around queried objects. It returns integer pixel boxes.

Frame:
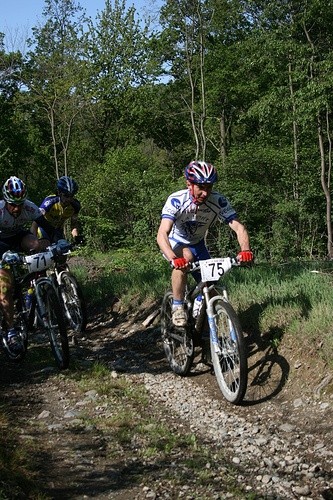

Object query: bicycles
[46,243,85,333]
[161,250,251,404]
[1,250,71,366]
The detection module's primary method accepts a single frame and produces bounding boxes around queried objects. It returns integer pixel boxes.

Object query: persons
[31,177,83,267]
[0,176,70,359]
[156,161,254,327]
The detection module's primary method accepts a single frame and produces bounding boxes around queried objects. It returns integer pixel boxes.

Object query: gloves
[72,236,83,246]
[58,239,72,254]
[2,250,20,265]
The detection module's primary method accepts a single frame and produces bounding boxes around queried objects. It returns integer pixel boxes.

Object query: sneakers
[24,295,32,308]
[201,344,213,365]
[171,304,188,326]
[6,328,22,354]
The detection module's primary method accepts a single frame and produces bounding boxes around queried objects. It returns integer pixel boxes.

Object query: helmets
[185,161,218,183]
[58,176,78,194]
[2,176,28,205]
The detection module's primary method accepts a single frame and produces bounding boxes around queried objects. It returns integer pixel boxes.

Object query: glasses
[63,194,74,198]
[9,203,25,206]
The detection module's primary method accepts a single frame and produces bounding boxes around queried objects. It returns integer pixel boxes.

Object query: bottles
[192,295,202,318]
[25,288,35,307]
